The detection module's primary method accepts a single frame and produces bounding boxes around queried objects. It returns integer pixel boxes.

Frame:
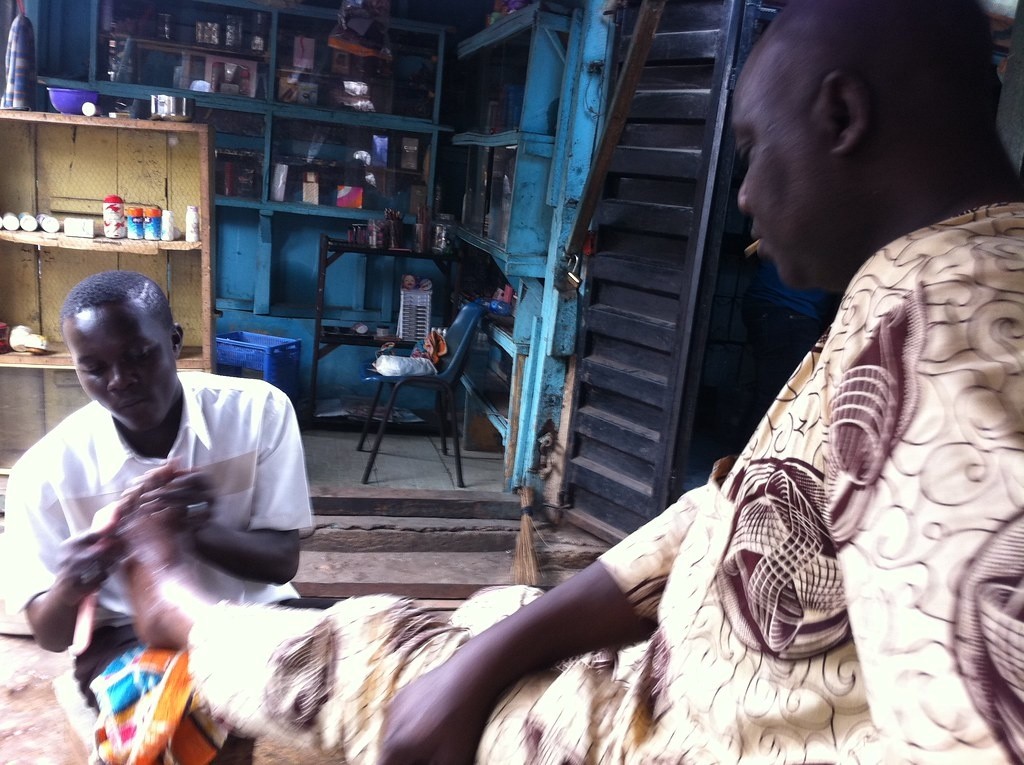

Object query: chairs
[356,301,486,489]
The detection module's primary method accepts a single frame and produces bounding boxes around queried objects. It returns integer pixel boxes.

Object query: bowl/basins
[46,88,100,115]
[150,94,196,122]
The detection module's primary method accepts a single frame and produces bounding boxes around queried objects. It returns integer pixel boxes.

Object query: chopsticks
[416,203,427,224]
[384,208,404,220]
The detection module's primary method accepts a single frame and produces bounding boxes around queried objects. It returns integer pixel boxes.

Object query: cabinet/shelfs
[25,0,449,322]
[302,232,463,438]
[0,110,214,498]
[446,0,587,494]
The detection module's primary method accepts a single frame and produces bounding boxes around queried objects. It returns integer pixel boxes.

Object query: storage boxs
[216,331,301,386]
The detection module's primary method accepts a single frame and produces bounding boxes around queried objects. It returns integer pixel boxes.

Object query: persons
[6,271,312,712]
[120,0,1024,765]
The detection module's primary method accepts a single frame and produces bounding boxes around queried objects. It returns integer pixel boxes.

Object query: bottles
[487,299,511,316]
[144,208,161,240]
[185,206,199,243]
[161,210,174,241]
[127,206,144,240]
[102,194,126,238]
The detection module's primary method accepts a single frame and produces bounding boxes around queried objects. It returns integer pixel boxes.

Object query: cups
[385,219,404,249]
[412,223,430,253]
